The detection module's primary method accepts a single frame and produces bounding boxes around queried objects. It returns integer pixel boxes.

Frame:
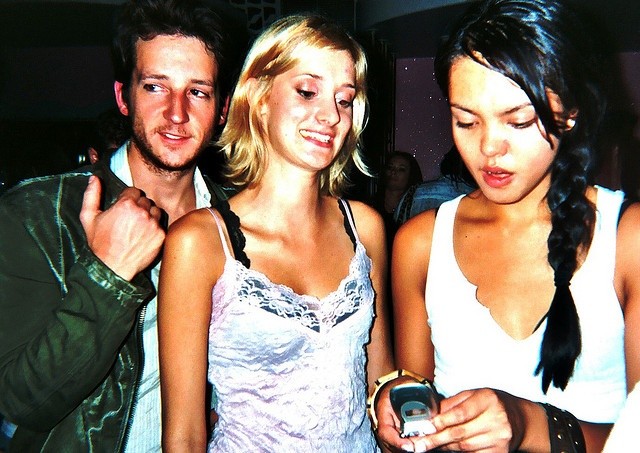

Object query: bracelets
[536,396,585,453]
[364,369,441,429]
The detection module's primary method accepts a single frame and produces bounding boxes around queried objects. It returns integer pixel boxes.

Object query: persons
[0,21,239,453]
[366,151,423,233]
[389,144,477,234]
[157,11,396,453]
[378,1,639,452]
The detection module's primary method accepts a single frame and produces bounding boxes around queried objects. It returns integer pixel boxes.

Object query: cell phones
[389,382,440,438]
